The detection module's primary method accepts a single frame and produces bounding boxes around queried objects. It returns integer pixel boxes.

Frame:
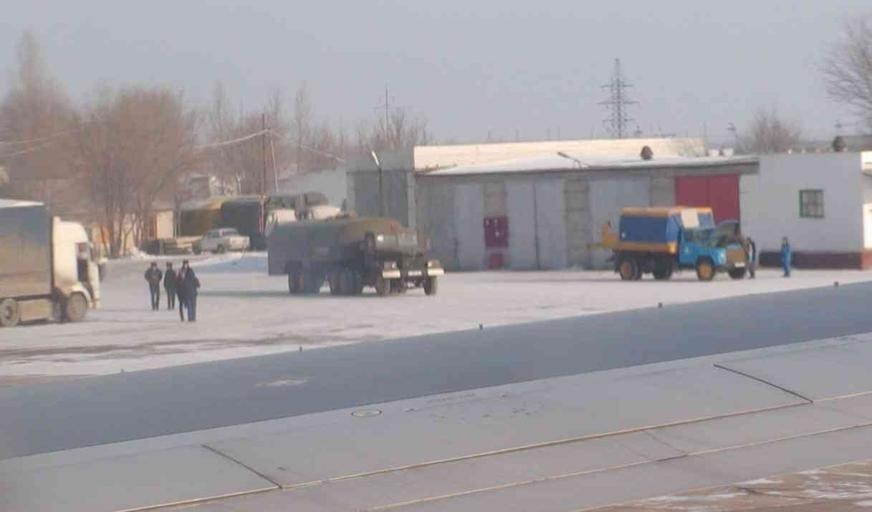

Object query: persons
[175,260,190,322]
[180,267,201,324]
[777,236,792,279]
[743,237,757,280]
[163,261,178,309]
[142,260,162,310]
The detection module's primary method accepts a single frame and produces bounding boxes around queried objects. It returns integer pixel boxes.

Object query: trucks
[0,198,103,329]
[598,204,755,282]
[265,215,445,298]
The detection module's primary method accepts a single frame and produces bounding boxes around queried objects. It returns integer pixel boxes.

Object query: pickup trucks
[192,228,251,255]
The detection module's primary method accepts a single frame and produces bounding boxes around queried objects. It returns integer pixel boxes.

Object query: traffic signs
[370,151,383,215]
